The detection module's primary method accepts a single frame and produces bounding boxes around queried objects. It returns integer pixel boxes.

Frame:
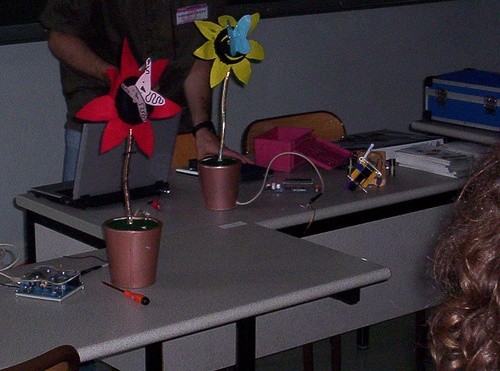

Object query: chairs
[241,111,347,155]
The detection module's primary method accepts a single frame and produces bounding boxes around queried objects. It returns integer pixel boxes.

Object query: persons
[46,0,255,183]
[424,145,500,371]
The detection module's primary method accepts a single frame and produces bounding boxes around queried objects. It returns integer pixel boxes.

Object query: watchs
[192,120,216,135]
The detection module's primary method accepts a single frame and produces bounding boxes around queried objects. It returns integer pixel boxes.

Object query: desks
[0,222,393,370]
[14,135,486,370]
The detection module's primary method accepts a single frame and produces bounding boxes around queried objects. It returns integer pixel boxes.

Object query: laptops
[31,112,181,209]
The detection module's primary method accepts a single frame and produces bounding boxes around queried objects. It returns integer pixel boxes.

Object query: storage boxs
[424,67,500,132]
[254,126,353,174]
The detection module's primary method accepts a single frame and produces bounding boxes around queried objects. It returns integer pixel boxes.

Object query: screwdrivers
[101,281,150,306]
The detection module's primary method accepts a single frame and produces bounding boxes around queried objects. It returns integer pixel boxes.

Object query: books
[393,143,490,179]
